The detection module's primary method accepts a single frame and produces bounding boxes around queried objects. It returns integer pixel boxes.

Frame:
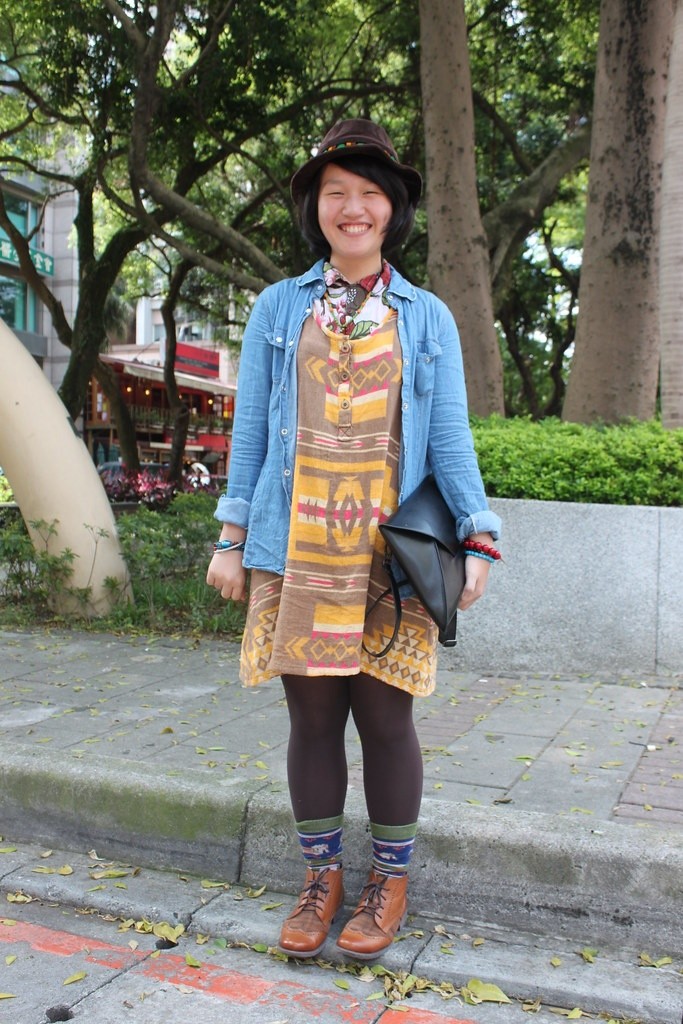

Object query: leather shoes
[279,864,345,958]
[336,869,409,960]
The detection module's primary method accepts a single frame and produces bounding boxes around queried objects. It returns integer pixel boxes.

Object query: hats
[291,119,422,206]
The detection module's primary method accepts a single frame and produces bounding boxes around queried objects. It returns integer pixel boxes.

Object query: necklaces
[324,287,372,333]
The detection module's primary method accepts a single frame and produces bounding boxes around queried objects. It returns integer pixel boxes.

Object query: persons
[205,117,503,961]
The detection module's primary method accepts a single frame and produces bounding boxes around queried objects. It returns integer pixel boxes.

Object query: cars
[99,461,173,488]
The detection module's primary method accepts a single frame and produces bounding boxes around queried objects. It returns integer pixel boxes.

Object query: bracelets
[463,538,501,563]
[212,539,244,553]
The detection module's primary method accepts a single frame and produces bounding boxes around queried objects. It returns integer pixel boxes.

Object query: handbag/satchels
[361,474,472,658]
